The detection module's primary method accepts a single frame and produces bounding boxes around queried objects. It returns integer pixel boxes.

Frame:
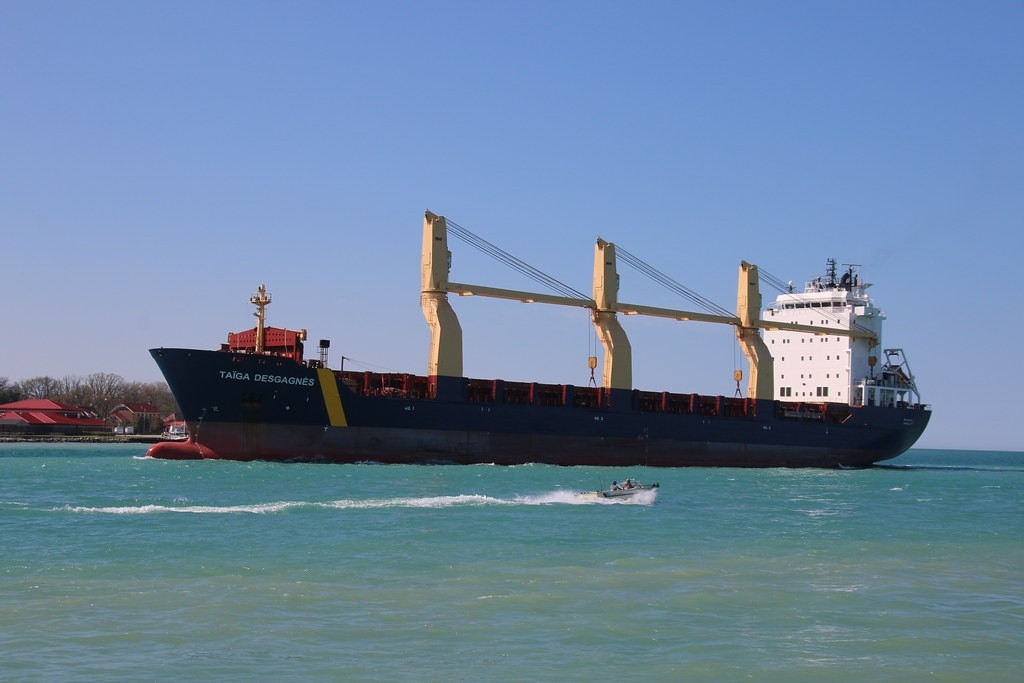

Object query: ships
[144,209,933,471]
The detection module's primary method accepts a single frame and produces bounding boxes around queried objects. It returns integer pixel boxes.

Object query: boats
[597,482,660,499]
[161,419,188,442]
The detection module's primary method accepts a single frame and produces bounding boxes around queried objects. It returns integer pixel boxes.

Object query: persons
[623,478,634,490]
[611,480,623,491]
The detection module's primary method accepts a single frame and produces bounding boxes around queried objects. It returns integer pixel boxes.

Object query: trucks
[114,426,121,435]
[125,427,134,435]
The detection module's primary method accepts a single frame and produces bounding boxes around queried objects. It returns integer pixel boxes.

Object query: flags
[162,413,176,422]
